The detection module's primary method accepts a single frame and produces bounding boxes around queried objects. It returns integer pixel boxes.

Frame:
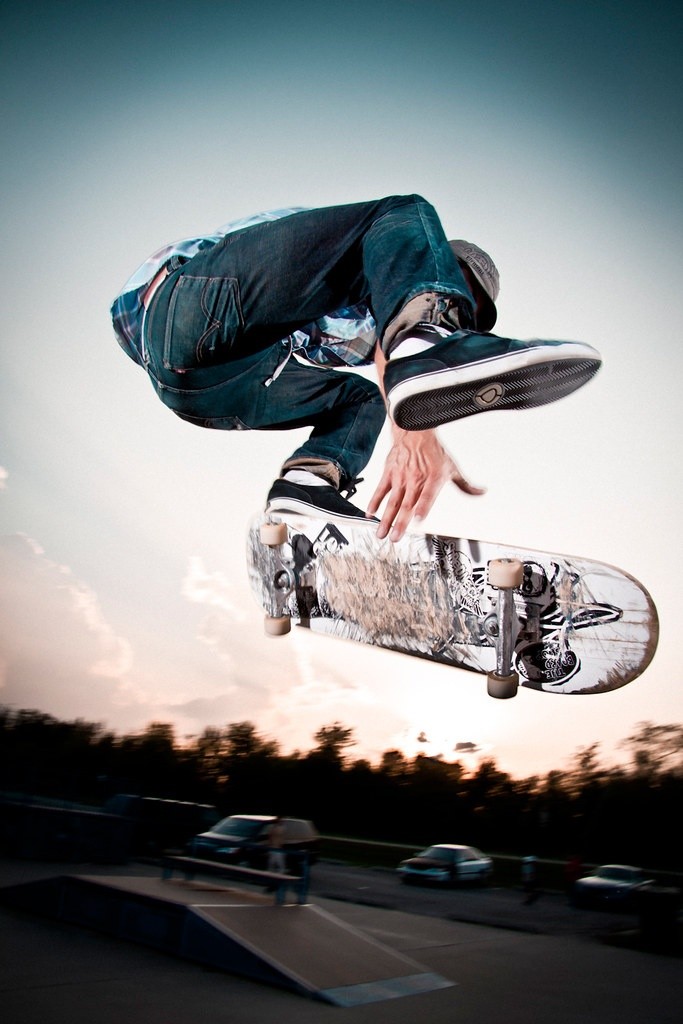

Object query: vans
[94,794,222,853]
[186,815,323,877]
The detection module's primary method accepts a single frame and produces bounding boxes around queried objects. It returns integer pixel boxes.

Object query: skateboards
[245,510,659,698]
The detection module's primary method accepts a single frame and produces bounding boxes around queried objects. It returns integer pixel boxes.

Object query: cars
[394,843,495,889]
[570,865,655,910]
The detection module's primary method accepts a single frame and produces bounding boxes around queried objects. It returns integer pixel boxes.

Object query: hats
[448,240,500,333]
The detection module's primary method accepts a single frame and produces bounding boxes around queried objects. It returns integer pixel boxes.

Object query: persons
[109,193,605,544]
[264,815,288,873]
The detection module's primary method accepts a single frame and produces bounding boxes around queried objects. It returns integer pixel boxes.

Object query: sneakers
[265,478,380,525]
[385,329,602,430]
